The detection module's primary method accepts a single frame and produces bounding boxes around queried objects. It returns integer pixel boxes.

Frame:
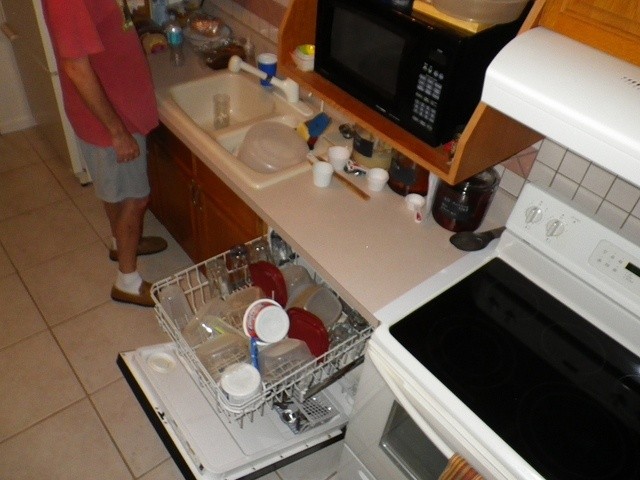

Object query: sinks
[210,114,339,189]
[152,68,303,133]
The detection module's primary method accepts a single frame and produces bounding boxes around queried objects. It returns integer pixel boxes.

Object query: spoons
[404,193,425,222]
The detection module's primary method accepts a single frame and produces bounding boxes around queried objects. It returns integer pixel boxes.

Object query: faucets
[229,55,299,103]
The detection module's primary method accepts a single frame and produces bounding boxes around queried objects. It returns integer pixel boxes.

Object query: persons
[41,0,168,307]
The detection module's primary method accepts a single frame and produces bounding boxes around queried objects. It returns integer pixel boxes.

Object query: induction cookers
[373,254,640,480]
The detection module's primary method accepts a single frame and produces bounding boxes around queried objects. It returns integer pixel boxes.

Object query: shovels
[308,154,370,200]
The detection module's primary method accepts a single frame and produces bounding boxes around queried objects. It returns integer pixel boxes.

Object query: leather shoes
[110,236,167,261]
[111,280,160,306]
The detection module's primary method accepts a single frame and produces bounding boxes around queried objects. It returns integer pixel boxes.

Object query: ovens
[343,338,521,480]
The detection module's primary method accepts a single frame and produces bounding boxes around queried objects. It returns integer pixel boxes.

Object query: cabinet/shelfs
[278,0,547,187]
[146,121,265,288]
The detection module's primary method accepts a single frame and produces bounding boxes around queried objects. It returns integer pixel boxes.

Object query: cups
[257,54,277,86]
[147,234,296,324]
[388,148,428,196]
[366,167,388,192]
[432,168,501,232]
[328,147,351,171]
[213,94,230,128]
[313,162,333,188]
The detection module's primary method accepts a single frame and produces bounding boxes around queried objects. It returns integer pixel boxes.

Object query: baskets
[150,234,373,428]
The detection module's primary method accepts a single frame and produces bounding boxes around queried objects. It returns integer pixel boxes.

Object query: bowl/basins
[182,15,232,51]
[291,40,314,74]
[184,266,339,415]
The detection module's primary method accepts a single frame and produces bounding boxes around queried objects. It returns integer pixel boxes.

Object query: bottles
[166,15,183,68]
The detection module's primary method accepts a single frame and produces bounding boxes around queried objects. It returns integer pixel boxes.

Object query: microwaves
[314,0,534,147]
